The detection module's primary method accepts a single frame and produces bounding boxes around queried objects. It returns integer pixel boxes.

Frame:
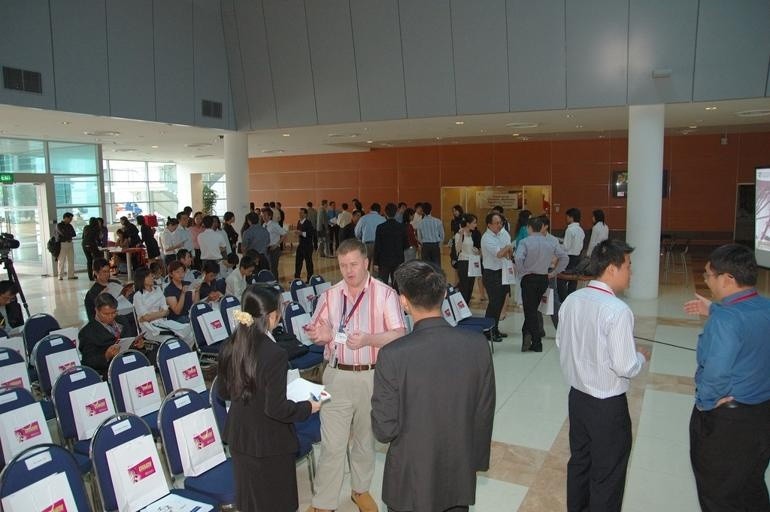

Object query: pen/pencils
[311,393,318,402]
[1,317,5,325]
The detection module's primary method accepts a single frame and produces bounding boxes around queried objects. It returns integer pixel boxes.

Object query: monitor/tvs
[612,170,668,199]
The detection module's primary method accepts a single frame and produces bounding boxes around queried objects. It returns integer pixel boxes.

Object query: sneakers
[350,490,378,512]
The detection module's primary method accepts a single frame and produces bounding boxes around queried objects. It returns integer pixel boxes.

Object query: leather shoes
[522,333,542,352]
[486,331,506,342]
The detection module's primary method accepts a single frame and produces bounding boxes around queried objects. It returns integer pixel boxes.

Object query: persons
[556,238,651,511]
[78,293,144,370]
[305,238,407,512]
[306,199,366,257]
[85,249,262,344]
[56,212,78,280]
[354,201,445,294]
[250,202,284,252]
[450,205,609,353]
[289,207,316,282]
[0,280,25,340]
[81,206,286,282]
[369,259,495,512]
[616,173,627,197]
[683,242,770,512]
[218,284,320,512]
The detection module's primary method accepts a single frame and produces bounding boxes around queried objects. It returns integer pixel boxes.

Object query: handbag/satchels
[46,237,61,258]
[538,287,555,315]
[501,260,516,285]
[317,224,326,237]
[272,327,308,361]
[442,291,472,327]
[466,255,482,277]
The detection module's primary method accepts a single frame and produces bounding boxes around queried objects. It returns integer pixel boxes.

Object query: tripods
[0,258,31,324]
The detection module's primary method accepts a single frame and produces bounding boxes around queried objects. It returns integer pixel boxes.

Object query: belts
[334,364,376,371]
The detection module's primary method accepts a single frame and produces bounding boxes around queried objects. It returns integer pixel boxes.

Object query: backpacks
[448,238,458,266]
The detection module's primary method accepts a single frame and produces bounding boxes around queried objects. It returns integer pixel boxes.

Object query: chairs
[0,313,321,511]
[101,270,497,379]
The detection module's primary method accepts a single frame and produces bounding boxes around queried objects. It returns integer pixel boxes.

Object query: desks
[98,246,147,279]
[557,273,594,286]
